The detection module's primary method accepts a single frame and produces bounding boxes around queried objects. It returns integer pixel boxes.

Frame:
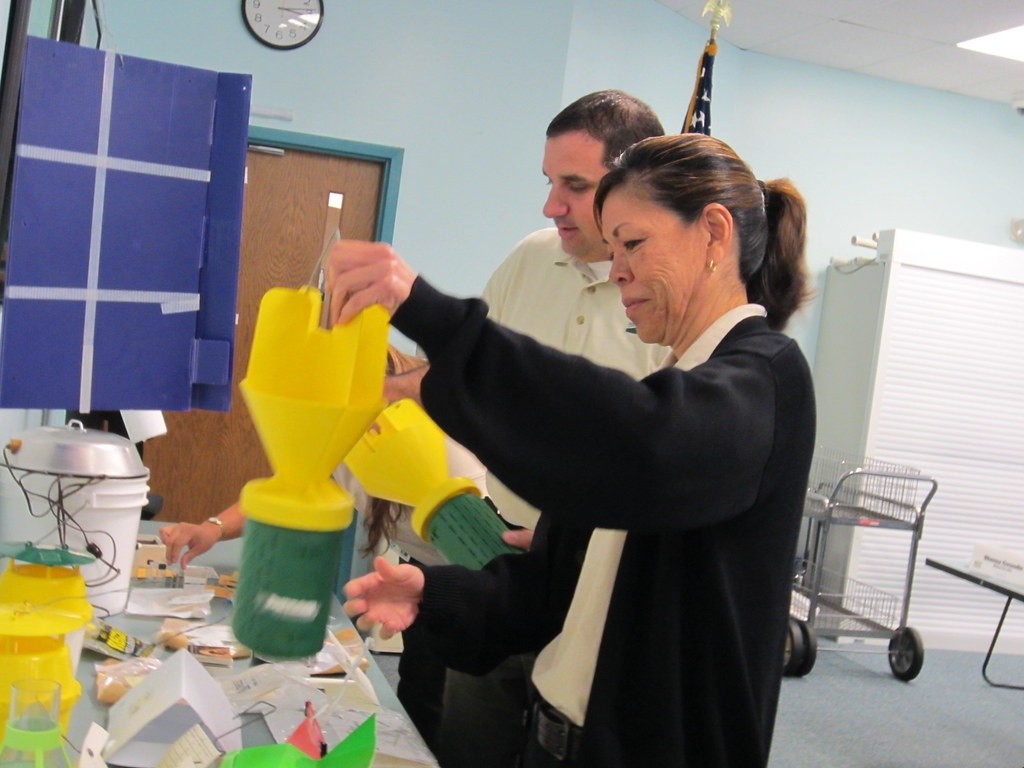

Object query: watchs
[208,517,226,540]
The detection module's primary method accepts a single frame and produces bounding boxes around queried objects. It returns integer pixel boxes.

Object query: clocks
[240,0,324,50]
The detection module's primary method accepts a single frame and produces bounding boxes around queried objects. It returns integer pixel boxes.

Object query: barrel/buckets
[0,462,151,617]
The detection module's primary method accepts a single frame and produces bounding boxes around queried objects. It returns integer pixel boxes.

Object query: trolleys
[776,467,938,681]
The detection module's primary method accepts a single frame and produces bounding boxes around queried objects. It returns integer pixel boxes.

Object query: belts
[527,699,580,759]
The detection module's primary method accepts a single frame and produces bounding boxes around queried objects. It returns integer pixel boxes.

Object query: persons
[321,133,819,768]
[385,91,673,768]
[158,346,488,754]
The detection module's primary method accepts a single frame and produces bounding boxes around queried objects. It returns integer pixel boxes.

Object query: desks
[68,517,440,768]
[926,554,1024,688]
